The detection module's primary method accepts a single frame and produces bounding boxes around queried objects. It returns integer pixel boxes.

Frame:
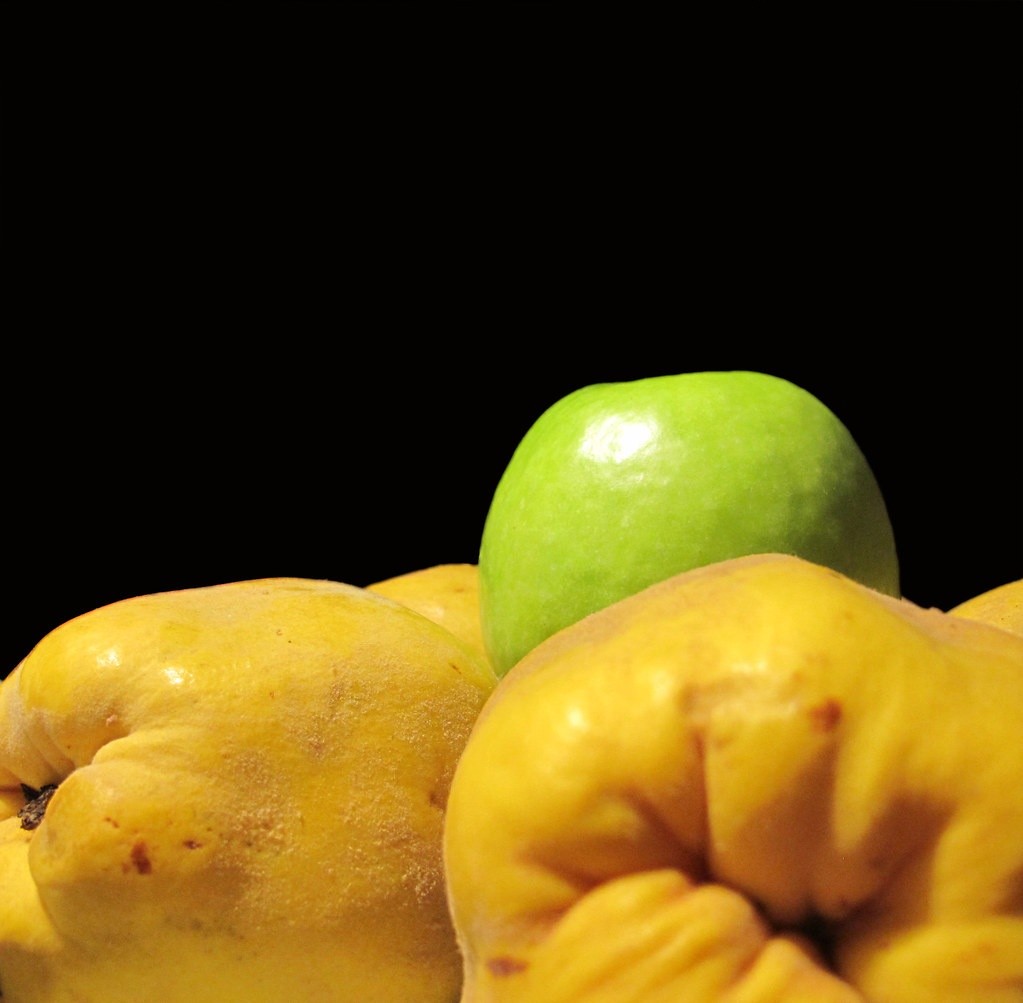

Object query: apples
[0,369,1023,1003]
